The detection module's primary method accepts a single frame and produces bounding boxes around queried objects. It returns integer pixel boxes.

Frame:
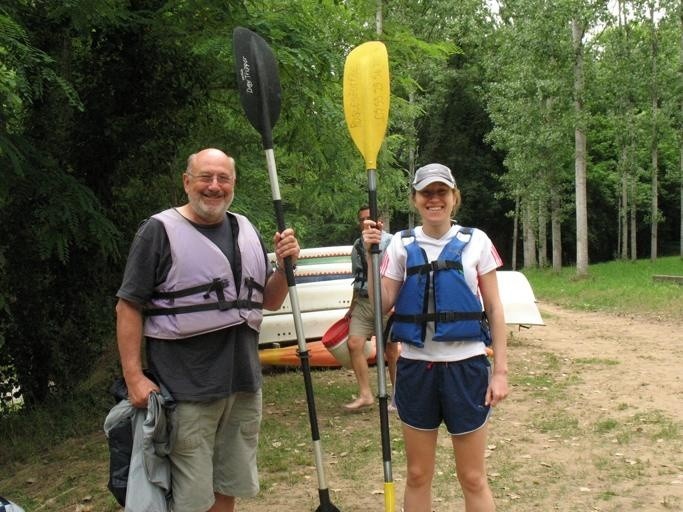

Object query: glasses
[188,172,235,184]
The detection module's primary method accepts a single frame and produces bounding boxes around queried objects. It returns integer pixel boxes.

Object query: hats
[412,163,455,190]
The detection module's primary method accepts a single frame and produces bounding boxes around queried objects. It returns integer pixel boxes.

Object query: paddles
[233,25,336,508]
[342,40,397,507]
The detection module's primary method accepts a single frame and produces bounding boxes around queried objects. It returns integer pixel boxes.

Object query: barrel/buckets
[321,317,375,370]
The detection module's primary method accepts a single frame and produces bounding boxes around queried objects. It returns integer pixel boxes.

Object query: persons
[341,205,401,411]
[361,163,508,512]
[114,148,299,512]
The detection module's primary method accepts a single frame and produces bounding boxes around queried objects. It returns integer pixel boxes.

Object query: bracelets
[275,261,296,275]
